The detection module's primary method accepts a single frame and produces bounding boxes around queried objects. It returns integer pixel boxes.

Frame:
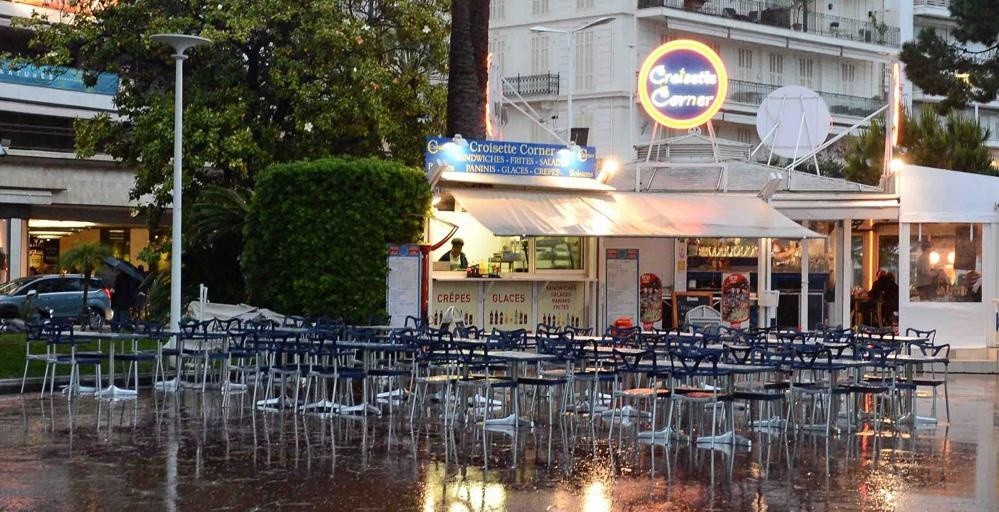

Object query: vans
[0,273,113,332]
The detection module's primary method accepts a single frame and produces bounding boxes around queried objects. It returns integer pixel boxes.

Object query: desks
[849,294,885,331]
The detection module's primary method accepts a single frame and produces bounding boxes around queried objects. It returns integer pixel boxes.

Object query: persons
[823,240,982,327]
[438,237,468,269]
[110,272,134,331]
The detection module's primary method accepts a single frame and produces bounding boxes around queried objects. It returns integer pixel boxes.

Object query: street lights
[530,14,618,148]
[147,31,215,372]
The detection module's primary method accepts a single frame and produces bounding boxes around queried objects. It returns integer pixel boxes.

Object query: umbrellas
[101,255,145,282]
